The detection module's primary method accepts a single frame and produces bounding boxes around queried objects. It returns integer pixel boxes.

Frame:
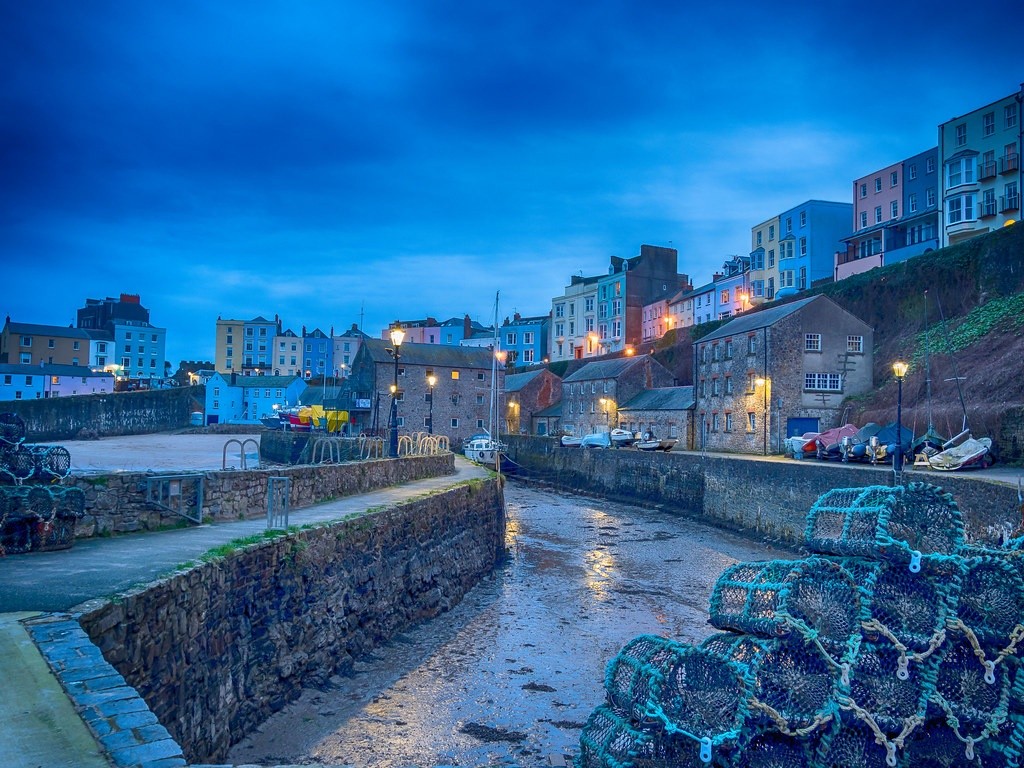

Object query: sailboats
[460,290,508,464]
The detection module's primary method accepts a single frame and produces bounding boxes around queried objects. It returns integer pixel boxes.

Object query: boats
[258,405,349,431]
[634,440,678,451]
[783,289,993,471]
[561,428,634,448]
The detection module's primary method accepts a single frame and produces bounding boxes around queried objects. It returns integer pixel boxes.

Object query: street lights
[385,320,406,458]
[892,348,909,471]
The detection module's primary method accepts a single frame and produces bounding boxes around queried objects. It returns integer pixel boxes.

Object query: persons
[644,428,653,442]
